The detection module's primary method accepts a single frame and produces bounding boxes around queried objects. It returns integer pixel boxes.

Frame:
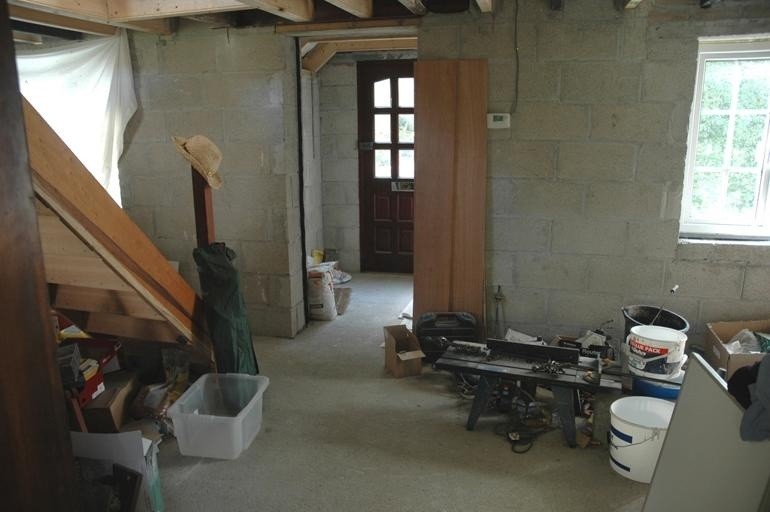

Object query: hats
[169,135,224,190]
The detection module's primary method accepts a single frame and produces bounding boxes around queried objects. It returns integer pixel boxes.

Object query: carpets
[332,288,352,315]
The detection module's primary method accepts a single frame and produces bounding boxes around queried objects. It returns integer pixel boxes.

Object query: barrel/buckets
[627,325,687,379]
[621,285,689,343]
[607,396,676,485]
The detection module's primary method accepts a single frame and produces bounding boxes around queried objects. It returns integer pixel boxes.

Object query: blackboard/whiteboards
[642,352,770,512]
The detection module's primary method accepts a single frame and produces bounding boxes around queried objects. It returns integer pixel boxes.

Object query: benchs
[435,339,624,448]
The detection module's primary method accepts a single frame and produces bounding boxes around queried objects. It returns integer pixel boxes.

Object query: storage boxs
[167,374,269,460]
[704,319,770,382]
[83,369,140,432]
[383,325,427,378]
